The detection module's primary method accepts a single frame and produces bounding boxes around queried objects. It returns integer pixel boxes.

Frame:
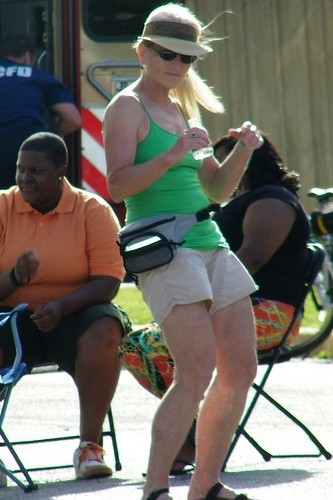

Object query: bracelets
[10,268,30,287]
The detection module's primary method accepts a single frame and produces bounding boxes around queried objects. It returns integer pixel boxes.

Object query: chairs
[0,361,121,490]
[0,302,32,493]
[168,242,333,471]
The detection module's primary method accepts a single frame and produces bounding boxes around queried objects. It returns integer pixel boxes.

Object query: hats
[137,20,214,56]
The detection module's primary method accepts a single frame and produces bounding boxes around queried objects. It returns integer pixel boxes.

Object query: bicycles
[275,187,333,358]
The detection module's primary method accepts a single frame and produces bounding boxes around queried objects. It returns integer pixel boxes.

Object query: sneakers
[73,442,112,479]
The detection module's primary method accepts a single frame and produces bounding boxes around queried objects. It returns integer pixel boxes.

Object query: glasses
[145,44,198,65]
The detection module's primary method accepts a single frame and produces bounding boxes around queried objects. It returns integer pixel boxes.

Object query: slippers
[142,457,196,478]
[201,483,250,500]
[147,488,170,500]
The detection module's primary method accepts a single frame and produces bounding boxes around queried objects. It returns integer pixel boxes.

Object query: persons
[118,132,310,478]
[0,31,82,189]
[0,132,125,480]
[104,3,264,500]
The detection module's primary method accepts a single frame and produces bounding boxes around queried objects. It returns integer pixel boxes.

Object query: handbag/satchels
[116,203,223,273]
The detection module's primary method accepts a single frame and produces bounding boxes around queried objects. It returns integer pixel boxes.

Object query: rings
[191,132,196,138]
